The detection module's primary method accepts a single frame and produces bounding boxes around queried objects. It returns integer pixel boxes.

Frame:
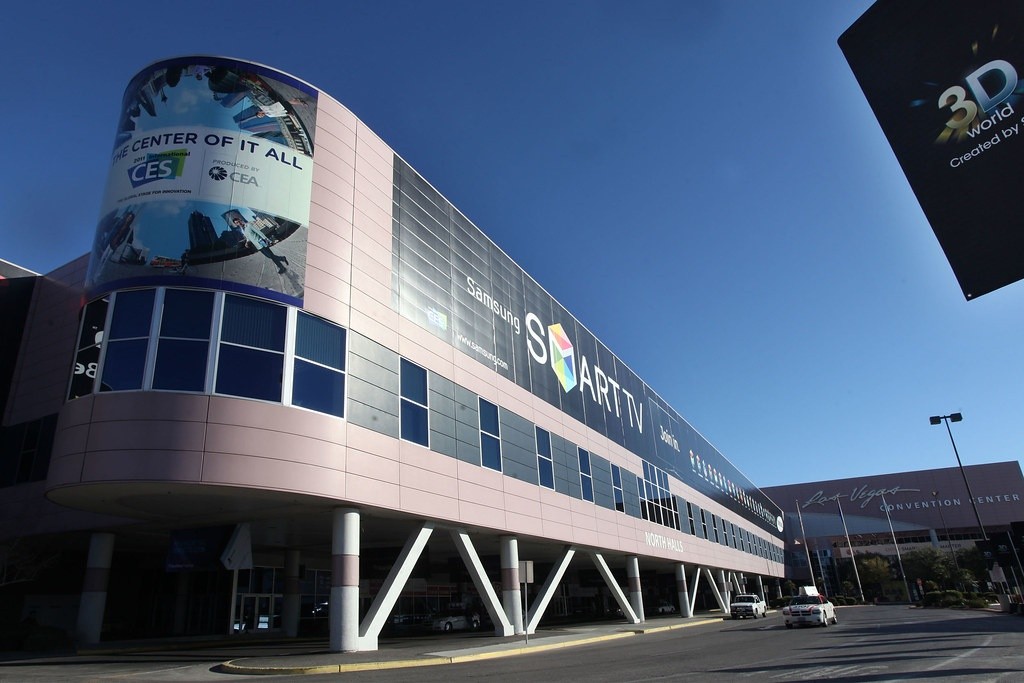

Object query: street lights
[930,413,1006,594]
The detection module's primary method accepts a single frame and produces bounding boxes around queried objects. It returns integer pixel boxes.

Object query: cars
[649,600,675,614]
[422,608,481,633]
[782,596,838,626]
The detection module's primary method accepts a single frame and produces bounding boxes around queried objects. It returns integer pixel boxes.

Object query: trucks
[604,595,632,619]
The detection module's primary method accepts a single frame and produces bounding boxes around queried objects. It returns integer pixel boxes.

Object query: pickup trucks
[730,595,767,619]
